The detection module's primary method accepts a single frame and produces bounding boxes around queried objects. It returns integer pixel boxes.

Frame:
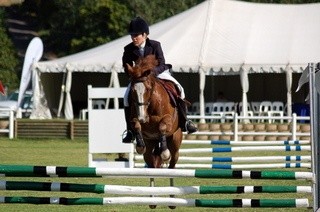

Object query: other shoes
[181,126,197,132]
[122,133,133,143]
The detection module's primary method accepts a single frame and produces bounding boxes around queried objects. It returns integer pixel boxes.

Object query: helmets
[128,17,149,35]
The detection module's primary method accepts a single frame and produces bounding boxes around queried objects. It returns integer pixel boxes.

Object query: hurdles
[0,163,315,208]
[87,84,312,169]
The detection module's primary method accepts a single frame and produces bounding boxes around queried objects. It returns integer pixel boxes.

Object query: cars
[0,90,33,119]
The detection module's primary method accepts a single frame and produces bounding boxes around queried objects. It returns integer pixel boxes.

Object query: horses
[125,54,183,210]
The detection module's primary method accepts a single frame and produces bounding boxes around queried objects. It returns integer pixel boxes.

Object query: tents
[28,0,320,123]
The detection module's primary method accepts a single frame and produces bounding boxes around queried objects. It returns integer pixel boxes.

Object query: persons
[122,17,198,143]
[216,91,227,102]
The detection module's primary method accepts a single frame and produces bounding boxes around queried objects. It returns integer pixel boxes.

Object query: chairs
[80,100,285,123]
